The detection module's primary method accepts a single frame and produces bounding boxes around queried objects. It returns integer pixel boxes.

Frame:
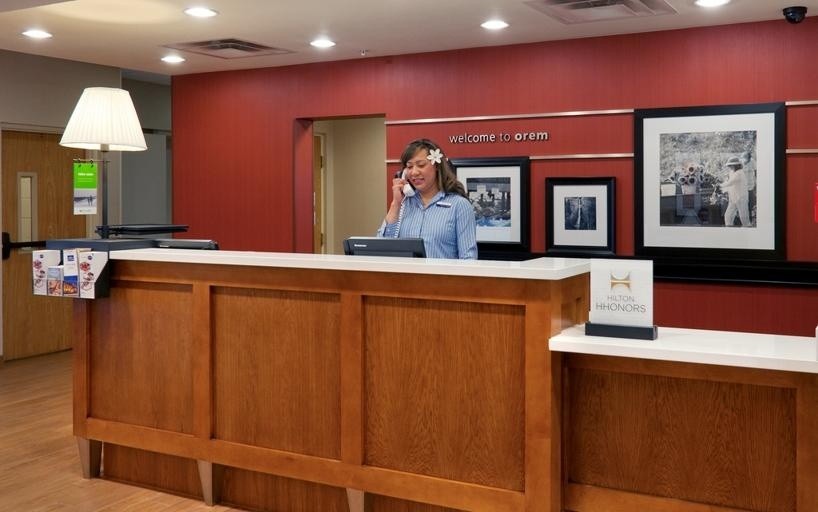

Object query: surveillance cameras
[782,6,807,23]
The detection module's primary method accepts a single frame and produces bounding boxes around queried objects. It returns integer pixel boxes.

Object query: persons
[376,139,478,260]
[714,157,753,227]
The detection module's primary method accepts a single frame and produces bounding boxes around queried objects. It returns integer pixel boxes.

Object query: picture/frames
[546,176,615,256]
[449,157,530,257]
[633,102,787,264]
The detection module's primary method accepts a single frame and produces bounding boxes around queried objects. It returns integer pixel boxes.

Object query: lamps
[46,86,154,250]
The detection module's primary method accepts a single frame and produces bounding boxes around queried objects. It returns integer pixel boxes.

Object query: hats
[725,157,742,165]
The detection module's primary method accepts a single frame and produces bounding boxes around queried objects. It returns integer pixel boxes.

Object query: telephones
[400,168,415,197]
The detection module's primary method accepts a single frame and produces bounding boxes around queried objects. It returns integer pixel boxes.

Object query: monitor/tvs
[344,236,427,259]
